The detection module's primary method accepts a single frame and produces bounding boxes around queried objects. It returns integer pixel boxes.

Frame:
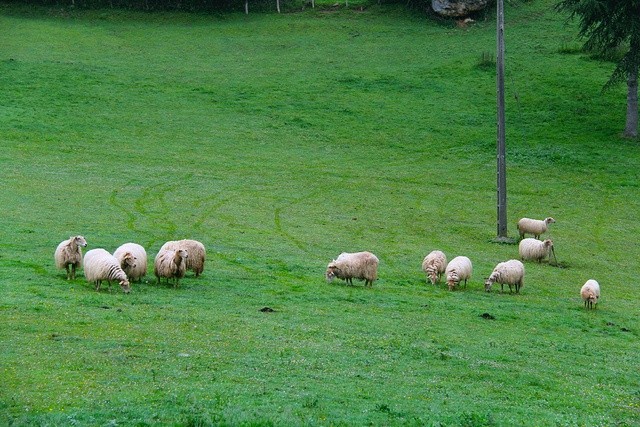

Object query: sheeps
[519,238,553,263]
[581,279,600,310]
[83,248,130,294]
[446,255,472,291]
[422,250,447,284]
[154,248,188,286]
[159,239,205,278]
[485,259,525,293]
[113,243,147,282]
[517,217,556,239]
[55,235,87,280]
[327,251,380,287]
